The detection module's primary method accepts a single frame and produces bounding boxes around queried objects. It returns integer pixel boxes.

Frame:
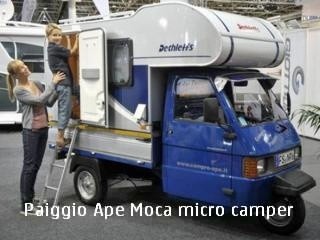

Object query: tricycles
[44,1,316,235]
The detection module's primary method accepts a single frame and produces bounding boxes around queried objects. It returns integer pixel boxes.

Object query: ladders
[39,126,77,212]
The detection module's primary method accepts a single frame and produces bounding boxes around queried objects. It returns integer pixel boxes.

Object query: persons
[46,22,79,154]
[7,59,67,216]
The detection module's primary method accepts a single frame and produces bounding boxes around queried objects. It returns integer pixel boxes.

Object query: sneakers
[57,138,71,147]
[20,207,41,215]
[33,199,47,206]
